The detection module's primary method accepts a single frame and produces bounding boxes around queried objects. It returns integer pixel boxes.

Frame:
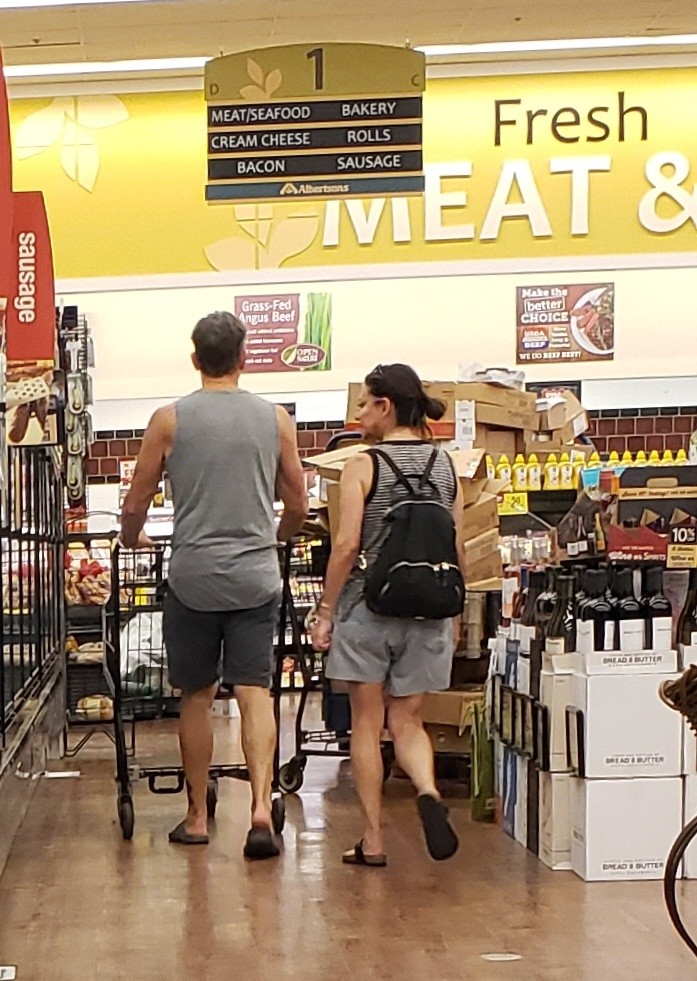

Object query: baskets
[68,663,161,722]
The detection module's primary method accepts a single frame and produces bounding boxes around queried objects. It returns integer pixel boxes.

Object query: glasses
[370,363,384,377]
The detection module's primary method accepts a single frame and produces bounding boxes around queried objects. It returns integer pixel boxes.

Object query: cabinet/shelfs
[67,527,166,756]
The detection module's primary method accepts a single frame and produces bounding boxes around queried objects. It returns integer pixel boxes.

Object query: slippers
[169,820,209,845]
[243,827,280,861]
[342,837,388,867]
[415,793,458,861]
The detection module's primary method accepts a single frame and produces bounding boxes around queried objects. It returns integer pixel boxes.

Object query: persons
[311,363,465,866]
[120,311,309,859]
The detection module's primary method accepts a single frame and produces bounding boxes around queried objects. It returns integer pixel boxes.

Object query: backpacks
[363,447,466,621]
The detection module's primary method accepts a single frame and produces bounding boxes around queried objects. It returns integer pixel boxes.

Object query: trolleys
[278,545,397,792]
[100,532,286,840]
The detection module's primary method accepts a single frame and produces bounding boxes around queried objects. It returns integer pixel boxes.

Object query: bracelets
[319,601,333,621]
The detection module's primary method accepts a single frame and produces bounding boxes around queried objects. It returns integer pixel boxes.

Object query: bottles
[566,513,606,556]
[485,449,689,491]
[512,560,697,651]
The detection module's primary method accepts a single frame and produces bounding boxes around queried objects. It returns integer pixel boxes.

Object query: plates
[570,287,614,355]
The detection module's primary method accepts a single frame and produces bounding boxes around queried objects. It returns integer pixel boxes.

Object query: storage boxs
[311,380,594,750]
[488,633,697,883]
